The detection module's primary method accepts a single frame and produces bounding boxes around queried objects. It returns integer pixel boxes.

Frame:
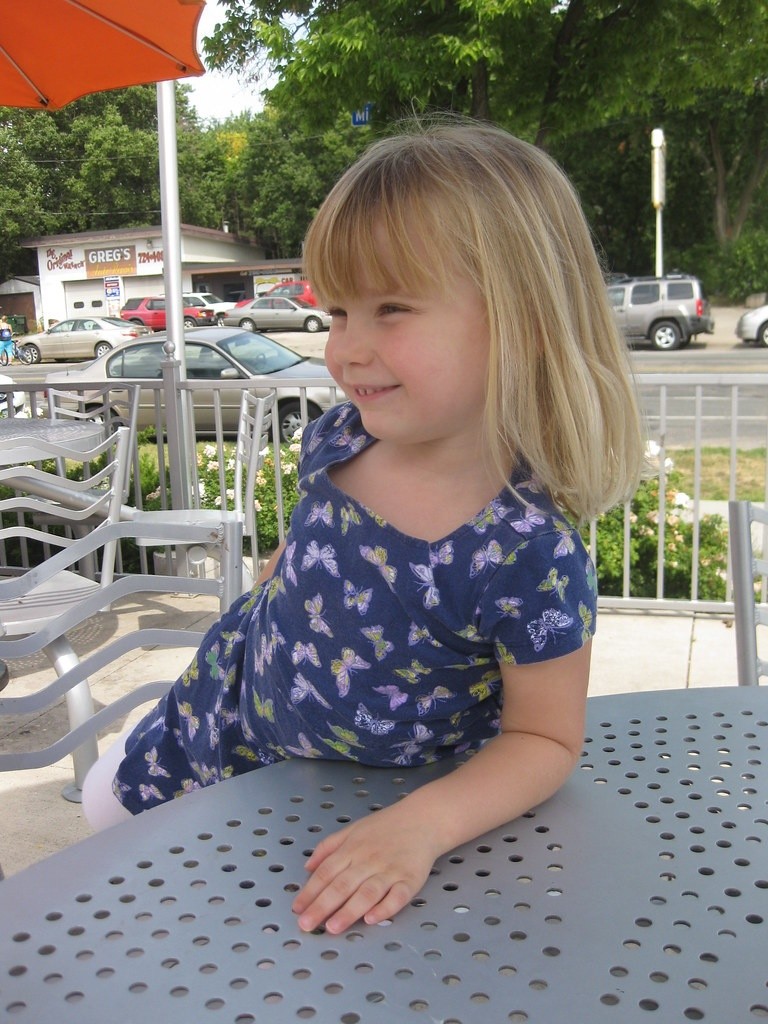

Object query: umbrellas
[0,0,207,113]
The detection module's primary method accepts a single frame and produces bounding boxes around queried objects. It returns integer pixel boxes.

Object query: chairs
[0,384,278,882]
[729,501,767,687]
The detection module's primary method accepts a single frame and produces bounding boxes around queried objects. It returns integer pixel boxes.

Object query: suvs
[607,274,715,349]
[158,292,236,322]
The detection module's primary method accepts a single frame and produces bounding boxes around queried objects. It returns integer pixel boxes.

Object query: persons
[84,123,643,936]
[0,307,12,366]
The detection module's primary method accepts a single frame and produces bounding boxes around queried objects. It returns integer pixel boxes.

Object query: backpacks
[0,329,11,341]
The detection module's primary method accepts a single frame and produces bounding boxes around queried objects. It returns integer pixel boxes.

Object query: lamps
[146,240,151,248]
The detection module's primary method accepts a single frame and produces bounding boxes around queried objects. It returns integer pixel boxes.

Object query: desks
[0,682,768,1024]
[0,419,106,690]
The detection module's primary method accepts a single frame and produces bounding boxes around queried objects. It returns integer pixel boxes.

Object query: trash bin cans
[8,315,26,336]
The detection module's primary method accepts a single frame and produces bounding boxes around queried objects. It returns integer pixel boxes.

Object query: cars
[224,297,332,334]
[235,280,319,308]
[45,323,348,439]
[17,316,154,365]
[736,302,768,350]
[0,374,25,418]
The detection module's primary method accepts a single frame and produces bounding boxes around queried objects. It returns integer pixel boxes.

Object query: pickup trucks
[120,296,214,333]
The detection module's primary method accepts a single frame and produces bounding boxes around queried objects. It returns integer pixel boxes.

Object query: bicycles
[0,331,34,367]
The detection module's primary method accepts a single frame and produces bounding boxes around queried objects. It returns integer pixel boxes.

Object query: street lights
[649,126,668,278]
